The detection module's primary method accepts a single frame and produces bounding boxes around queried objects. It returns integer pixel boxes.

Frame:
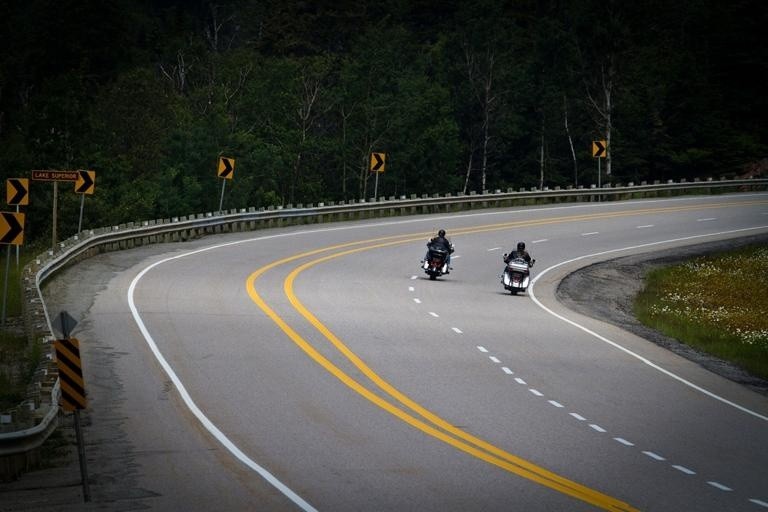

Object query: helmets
[517,243,525,249]
[439,230,445,237]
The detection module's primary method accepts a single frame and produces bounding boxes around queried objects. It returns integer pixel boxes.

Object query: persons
[500,242,533,278]
[421,229,452,276]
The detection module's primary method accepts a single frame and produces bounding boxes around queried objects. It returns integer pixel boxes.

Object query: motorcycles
[500,253,535,295]
[420,238,455,280]
